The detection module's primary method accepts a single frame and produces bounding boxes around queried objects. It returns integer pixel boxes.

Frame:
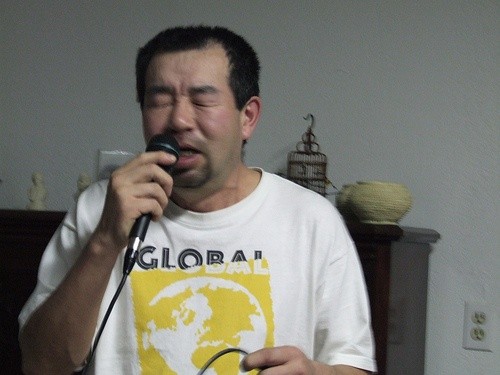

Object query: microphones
[122,134,180,276]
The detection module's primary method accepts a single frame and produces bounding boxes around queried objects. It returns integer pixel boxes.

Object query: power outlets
[463,302,494,351]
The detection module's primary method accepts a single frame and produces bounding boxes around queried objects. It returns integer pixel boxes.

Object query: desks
[0,209,441,375]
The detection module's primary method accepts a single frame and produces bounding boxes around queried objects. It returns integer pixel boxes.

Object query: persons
[28,173,47,202]
[15,24,378,375]
[76,173,91,190]
